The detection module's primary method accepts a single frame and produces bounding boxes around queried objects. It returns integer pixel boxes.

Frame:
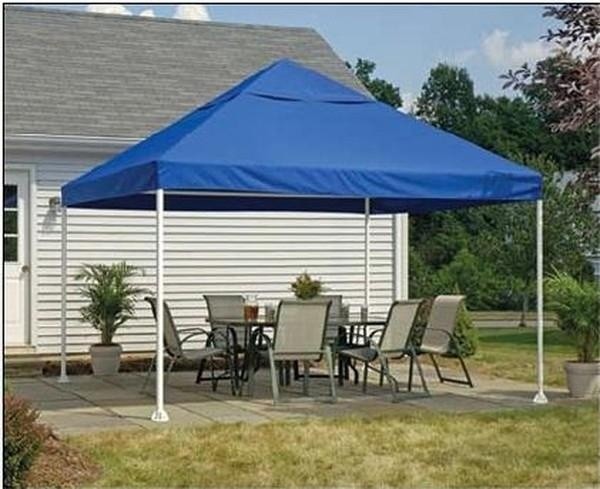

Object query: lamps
[48,193,62,213]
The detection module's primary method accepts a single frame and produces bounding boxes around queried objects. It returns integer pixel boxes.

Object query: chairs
[245,299,337,404]
[316,295,343,342]
[203,293,277,354]
[400,295,471,392]
[146,296,235,399]
[338,299,433,405]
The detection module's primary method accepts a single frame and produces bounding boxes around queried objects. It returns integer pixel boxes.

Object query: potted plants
[543,268,600,398]
[74,262,154,377]
[287,269,325,302]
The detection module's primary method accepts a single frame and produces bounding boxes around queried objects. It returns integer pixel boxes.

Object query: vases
[245,306,258,320]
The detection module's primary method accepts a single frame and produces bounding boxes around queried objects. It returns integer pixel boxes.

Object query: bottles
[243,295,259,320]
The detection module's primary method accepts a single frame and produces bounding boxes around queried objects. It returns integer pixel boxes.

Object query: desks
[205,317,388,397]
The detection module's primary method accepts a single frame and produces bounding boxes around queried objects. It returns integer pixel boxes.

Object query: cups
[264,302,276,322]
